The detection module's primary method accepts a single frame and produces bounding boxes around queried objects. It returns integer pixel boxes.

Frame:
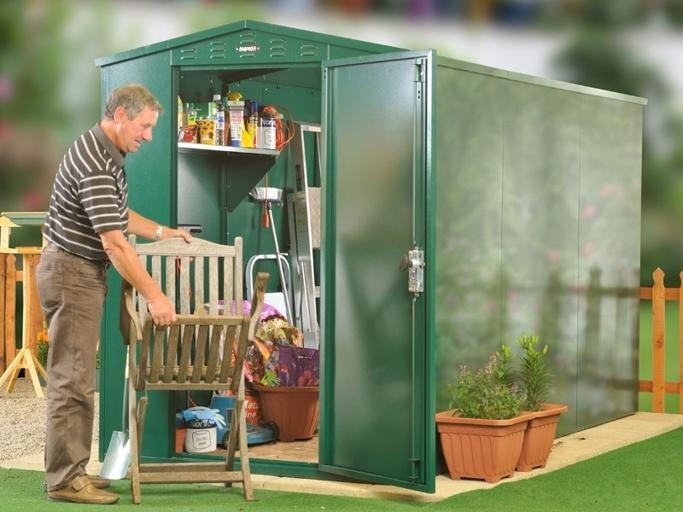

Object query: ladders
[286,120,321,351]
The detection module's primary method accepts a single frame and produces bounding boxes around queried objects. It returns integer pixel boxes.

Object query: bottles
[178,92,258,149]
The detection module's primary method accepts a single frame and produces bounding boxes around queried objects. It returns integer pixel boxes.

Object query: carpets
[1,422,682,512]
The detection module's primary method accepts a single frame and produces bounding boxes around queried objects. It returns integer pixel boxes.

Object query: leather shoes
[44,475,120,505]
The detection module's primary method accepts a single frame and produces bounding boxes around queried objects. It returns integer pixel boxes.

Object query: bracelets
[153,223,164,240]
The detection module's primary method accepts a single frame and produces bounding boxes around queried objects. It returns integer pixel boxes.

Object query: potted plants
[432,334,564,483]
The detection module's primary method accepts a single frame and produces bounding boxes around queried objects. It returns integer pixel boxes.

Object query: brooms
[250,188,295,326]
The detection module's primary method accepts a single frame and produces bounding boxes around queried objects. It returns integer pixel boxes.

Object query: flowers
[34,330,49,364]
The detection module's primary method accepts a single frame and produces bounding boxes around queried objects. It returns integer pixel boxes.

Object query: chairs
[119,233,270,505]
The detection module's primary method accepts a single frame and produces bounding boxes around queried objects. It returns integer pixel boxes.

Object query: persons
[32,83,196,505]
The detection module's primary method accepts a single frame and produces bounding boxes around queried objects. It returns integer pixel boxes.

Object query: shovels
[100,344,132,480]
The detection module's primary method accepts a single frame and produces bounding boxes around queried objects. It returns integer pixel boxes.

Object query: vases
[250,384,320,443]
